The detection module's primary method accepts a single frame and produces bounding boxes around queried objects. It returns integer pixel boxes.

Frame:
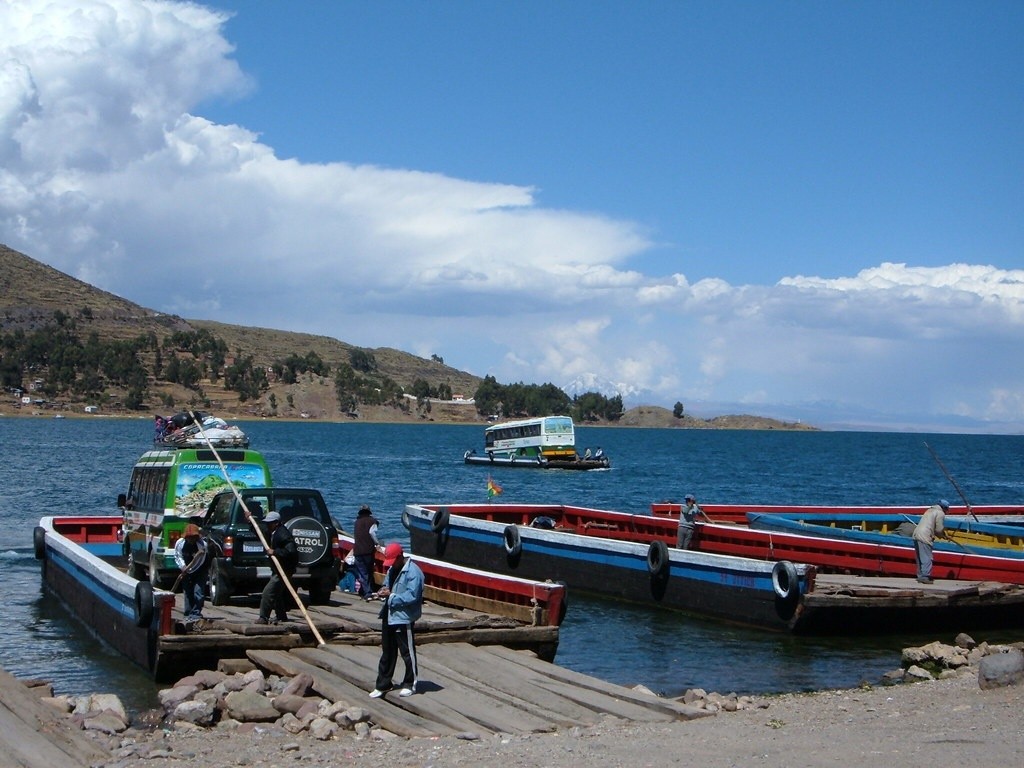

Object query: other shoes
[363,597,372,601]
[268,616,288,622]
[335,586,342,592]
[255,618,268,625]
[917,577,932,585]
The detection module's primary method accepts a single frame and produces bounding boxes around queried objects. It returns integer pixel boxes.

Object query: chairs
[238,503,264,523]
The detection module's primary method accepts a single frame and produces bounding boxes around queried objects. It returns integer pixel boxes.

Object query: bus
[484,414,576,460]
[115,436,275,591]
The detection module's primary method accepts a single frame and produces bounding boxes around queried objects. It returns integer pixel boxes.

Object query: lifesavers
[771,560,798,601]
[401,510,410,530]
[134,580,154,628]
[503,525,521,555]
[33,527,45,559]
[431,507,450,532]
[646,540,669,575]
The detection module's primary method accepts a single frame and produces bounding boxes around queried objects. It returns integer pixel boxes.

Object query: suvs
[190,486,342,607]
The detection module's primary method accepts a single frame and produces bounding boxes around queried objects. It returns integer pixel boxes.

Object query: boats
[31,513,571,687]
[401,501,1024,636]
[463,450,611,470]
[650,499,1024,561]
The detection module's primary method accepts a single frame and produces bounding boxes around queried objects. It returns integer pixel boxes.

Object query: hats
[180,524,200,538]
[938,501,950,509]
[685,494,695,501]
[382,543,402,566]
[358,505,372,514]
[261,511,280,522]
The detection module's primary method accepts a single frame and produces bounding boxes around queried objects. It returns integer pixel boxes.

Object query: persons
[352,504,383,599]
[368,544,425,699]
[595,447,604,462]
[676,494,714,550]
[244,511,299,623]
[174,523,222,621]
[913,500,952,584]
[584,447,591,460]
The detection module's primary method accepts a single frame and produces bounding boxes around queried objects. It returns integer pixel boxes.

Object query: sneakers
[369,687,393,698]
[399,688,413,697]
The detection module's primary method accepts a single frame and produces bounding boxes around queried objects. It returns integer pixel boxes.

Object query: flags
[488,480,502,497]
